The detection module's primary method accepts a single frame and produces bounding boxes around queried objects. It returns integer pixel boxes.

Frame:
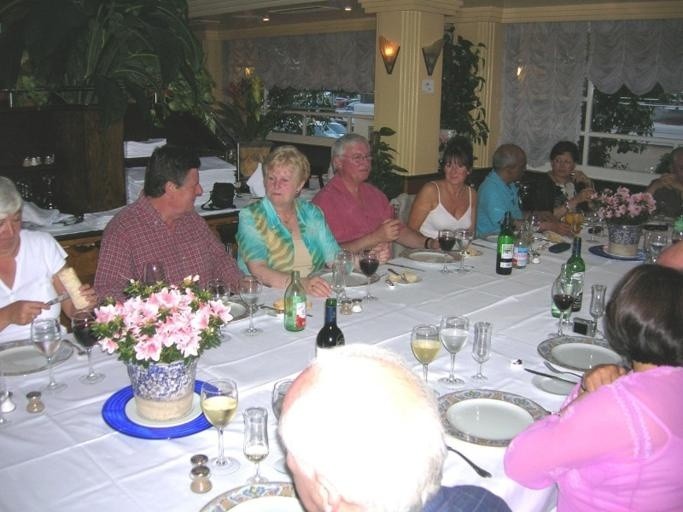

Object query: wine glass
[409,325,439,391]
[13,174,58,209]
[472,320,491,384]
[30,319,65,394]
[71,310,105,385]
[328,248,353,300]
[455,231,475,270]
[202,279,232,344]
[272,376,295,475]
[357,248,381,303]
[235,275,263,335]
[436,228,455,277]
[199,379,241,477]
[441,313,469,388]
[492,208,683,384]
[321,269,371,290]
[241,406,267,483]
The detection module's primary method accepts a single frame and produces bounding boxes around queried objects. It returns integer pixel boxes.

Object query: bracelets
[579,384,587,392]
[563,200,570,212]
[422,237,437,249]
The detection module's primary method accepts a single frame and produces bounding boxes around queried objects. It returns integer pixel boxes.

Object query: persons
[276,341,511,511]
[234,144,392,300]
[503,264,682,511]
[0,174,97,344]
[90,142,257,304]
[525,140,596,220]
[642,147,682,221]
[407,136,477,250]
[474,144,571,239]
[308,133,446,270]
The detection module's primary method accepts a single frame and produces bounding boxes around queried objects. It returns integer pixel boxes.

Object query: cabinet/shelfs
[265,89,374,188]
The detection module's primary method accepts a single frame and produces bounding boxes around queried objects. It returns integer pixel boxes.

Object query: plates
[0,337,72,376]
[229,297,250,326]
[403,246,460,267]
[201,482,301,512]
[266,305,279,319]
[437,389,549,450]
[532,370,577,397]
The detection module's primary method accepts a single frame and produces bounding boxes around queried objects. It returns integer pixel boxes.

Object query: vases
[606,223,642,256]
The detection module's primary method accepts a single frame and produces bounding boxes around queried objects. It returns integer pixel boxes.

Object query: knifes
[41,295,68,305]
[259,304,313,318]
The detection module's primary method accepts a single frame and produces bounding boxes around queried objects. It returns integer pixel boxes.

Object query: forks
[62,338,90,357]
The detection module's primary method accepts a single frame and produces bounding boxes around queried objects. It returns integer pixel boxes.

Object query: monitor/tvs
[236,140,273,193]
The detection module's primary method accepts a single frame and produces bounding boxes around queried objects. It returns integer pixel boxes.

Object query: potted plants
[204,73,298,178]
[0,0,218,217]
[438,22,488,176]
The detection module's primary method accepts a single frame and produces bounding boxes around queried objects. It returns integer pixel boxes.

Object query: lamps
[378,34,401,74]
[422,38,446,75]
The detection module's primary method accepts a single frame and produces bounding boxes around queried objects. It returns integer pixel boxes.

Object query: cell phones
[588,226,604,234]
[549,242,571,253]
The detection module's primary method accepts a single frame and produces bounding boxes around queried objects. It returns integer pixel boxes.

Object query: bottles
[18,152,55,166]
[24,391,44,413]
[189,453,212,495]
[0,391,15,413]
[283,271,305,331]
[313,296,345,352]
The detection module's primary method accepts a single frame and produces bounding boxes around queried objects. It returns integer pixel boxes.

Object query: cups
[582,178,593,189]
[141,261,168,287]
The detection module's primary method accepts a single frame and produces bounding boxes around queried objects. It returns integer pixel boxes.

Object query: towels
[17,135,266,230]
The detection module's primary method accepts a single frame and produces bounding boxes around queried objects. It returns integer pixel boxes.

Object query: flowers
[589,185,657,225]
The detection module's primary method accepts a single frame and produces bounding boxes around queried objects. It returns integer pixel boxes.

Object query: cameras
[572,317,596,337]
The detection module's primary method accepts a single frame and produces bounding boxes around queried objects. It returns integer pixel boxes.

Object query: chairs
[391,191,417,257]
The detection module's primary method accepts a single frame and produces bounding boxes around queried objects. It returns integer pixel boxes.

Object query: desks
[53,210,240,292]
